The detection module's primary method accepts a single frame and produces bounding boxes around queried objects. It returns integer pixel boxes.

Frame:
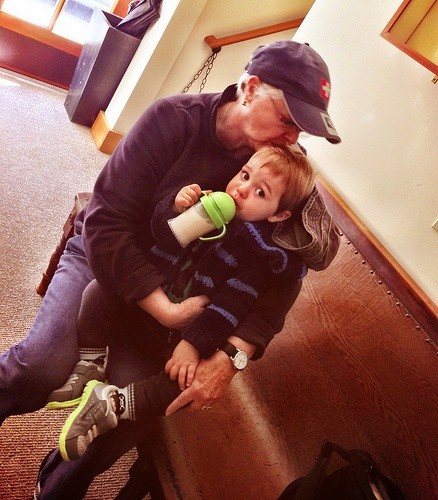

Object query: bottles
[167,192,236,248]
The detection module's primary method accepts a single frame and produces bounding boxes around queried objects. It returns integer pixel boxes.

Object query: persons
[47,142,315,463]
[0,40,344,500]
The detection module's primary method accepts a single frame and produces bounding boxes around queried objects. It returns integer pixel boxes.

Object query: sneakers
[58,379,120,462]
[45,358,120,411]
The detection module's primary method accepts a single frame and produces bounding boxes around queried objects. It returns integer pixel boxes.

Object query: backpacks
[277,439,406,500]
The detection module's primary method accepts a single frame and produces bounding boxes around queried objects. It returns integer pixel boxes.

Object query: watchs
[220,340,248,369]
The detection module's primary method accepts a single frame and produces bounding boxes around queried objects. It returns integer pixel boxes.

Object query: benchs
[36,160,438,500]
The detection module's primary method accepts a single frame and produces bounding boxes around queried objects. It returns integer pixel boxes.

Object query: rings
[202,406,211,411]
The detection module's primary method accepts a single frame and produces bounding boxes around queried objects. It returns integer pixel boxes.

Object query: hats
[243,40,342,144]
[271,185,344,272]
